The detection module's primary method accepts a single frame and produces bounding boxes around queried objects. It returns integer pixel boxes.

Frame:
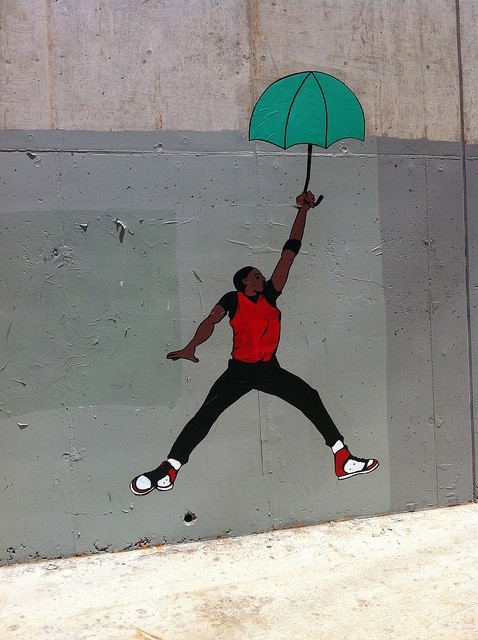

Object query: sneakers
[129,459,178,495]
[334,447,379,480]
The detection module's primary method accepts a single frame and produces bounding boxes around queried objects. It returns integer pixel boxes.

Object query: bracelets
[284,239,301,254]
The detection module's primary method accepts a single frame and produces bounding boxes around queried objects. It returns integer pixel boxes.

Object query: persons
[128,190,380,497]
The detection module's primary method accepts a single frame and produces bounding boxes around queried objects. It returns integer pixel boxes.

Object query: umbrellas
[247,69,366,208]
